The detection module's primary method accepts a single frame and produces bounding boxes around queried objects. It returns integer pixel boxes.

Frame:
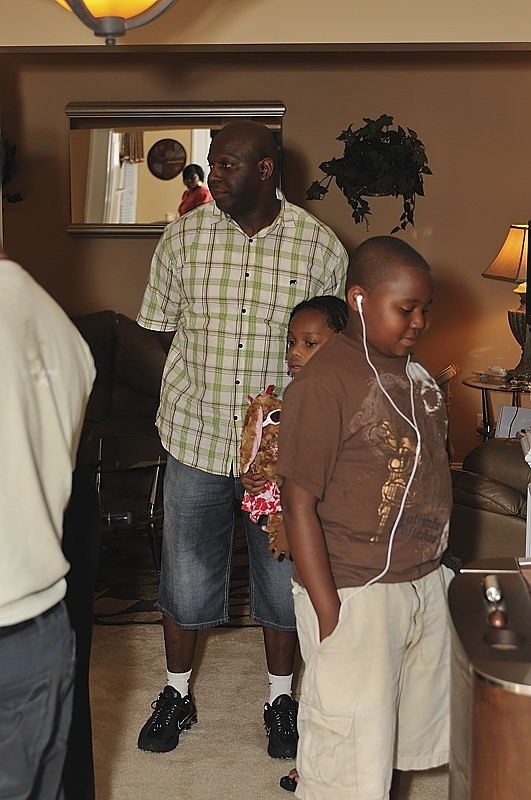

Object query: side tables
[461,376,530,443]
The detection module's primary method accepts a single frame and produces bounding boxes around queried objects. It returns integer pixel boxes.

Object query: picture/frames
[147,139,187,180]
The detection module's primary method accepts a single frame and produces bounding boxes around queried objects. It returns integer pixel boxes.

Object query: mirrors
[64,101,285,236]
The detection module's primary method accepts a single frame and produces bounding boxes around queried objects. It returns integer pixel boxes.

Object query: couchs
[68,309,177,499]
[451,438,531,563]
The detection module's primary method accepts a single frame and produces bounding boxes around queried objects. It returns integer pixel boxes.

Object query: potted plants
[304,113,434,236]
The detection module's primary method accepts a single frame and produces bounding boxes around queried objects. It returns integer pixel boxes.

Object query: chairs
[87,430,168,570]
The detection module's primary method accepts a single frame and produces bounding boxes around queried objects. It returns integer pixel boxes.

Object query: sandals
[280,775,299,791]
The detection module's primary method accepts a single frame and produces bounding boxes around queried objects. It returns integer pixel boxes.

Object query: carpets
[92,514,263,628]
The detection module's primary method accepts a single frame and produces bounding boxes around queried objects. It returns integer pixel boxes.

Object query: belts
[0,603,61,639]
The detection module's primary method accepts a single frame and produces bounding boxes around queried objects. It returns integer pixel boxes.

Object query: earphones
[356,294,363,314]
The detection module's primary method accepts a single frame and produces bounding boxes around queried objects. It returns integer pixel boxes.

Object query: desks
[446,554,531,800]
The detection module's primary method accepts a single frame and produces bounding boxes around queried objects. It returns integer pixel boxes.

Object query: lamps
[53,0,180,47]
[481,223,531,350]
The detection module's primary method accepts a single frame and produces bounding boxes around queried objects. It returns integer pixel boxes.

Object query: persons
[1,257,97,800]
[277,235,455,800]
[279,295,350,792]
[178,164,213,217]
[136,119,350,759]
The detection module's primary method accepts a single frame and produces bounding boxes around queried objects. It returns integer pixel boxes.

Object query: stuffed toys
[239,384,282,550]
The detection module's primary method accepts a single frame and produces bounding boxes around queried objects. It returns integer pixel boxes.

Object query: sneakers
[138,685,198,753]
[263,694,299,759]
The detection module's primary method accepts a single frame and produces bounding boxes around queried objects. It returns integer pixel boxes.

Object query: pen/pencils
[459,569,520,574]
